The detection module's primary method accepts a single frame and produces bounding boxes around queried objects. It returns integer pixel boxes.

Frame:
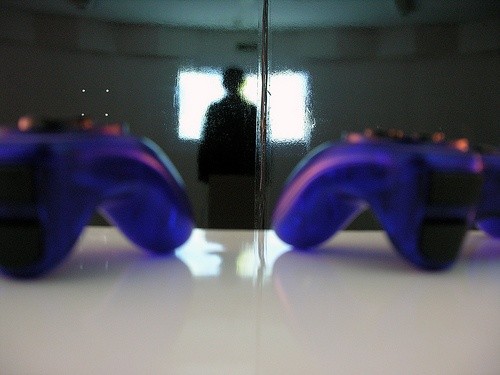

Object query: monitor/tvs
[177,66,312,147]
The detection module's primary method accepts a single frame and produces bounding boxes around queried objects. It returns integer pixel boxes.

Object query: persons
[194,64,268,228]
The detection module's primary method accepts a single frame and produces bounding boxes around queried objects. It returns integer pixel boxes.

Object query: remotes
[271,133,500,271]
[2,133,190,279]
